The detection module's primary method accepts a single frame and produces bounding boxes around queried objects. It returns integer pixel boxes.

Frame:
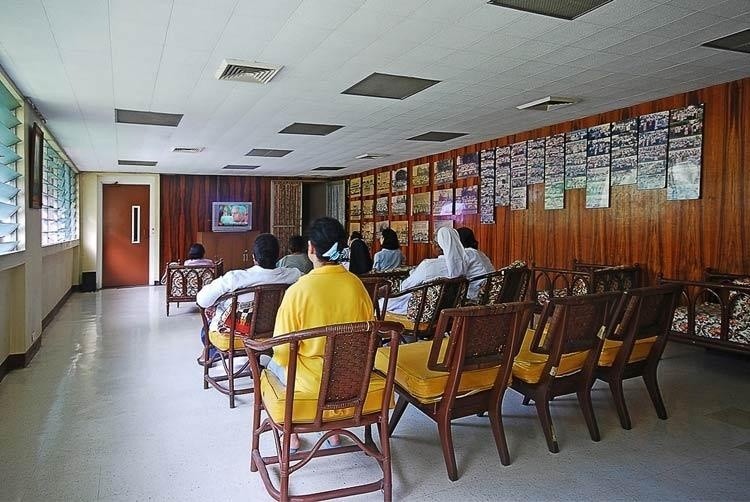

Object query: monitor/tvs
[212,202,253,233]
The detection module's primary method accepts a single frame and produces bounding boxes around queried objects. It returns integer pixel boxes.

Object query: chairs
[356,234,693,363]
[203,283,293,408]
[167,259,223,317]
[588,282,682,430]
[507,291,620,453]
[372,302,536,481]
[242,320,406,502]
[654,265,750,357]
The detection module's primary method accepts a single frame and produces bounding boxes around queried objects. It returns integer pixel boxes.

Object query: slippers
[279,433,297,454]
[197,358,216,367]
[319,432,342,448]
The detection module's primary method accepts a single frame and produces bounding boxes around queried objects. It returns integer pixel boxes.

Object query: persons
[260,215,375,392]
[349,238,373,274]
[275,234,313,274]
[341,230,365,257]
[197,232,306,368]
[457,226,497,302]
[374,225,469,347]
[373,227,407,271]
[184,243,214,266]
